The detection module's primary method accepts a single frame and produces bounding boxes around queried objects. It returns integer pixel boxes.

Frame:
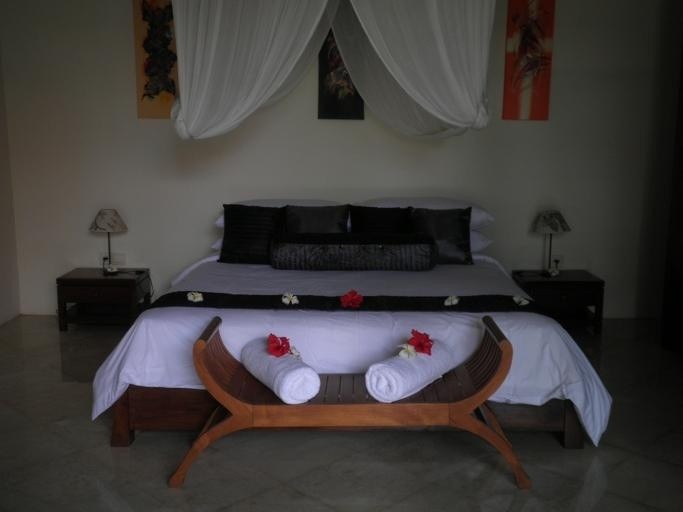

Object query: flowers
[186,291,205,303]
[281,291,300,305]
[407,328,435,356]
[443,294,460,306]
[396,342,418,360]
[267,333,300,359]
[340,289,365,308]
[513,293,530,305]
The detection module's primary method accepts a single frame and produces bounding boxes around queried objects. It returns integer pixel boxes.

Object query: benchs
[166,315,533,490]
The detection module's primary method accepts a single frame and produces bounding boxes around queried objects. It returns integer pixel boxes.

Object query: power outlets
[99,252,126,267]
[551,255,564,269]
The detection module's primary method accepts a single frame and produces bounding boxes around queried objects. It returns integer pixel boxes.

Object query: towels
[361,335,458,405]
[239,332,323,405]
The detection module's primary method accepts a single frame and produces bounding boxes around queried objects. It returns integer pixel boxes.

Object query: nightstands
[56,268,151,382]
[512,270,604,376]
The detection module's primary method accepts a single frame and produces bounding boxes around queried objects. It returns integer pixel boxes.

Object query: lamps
[88,209,129,274]
[527,210,571,276]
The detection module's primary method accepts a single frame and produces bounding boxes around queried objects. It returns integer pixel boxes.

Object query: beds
[92,255,613,449]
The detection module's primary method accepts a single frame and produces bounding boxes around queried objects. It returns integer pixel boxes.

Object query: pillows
[352,198,494,230]
[212,237,222,250]
[215,199,350,229]
[349,206,411,245]
[217,204,289,265]
[265,235,437,272]
[286,204,350,245]
[410,207,473,265]
[471,231,495,253]
